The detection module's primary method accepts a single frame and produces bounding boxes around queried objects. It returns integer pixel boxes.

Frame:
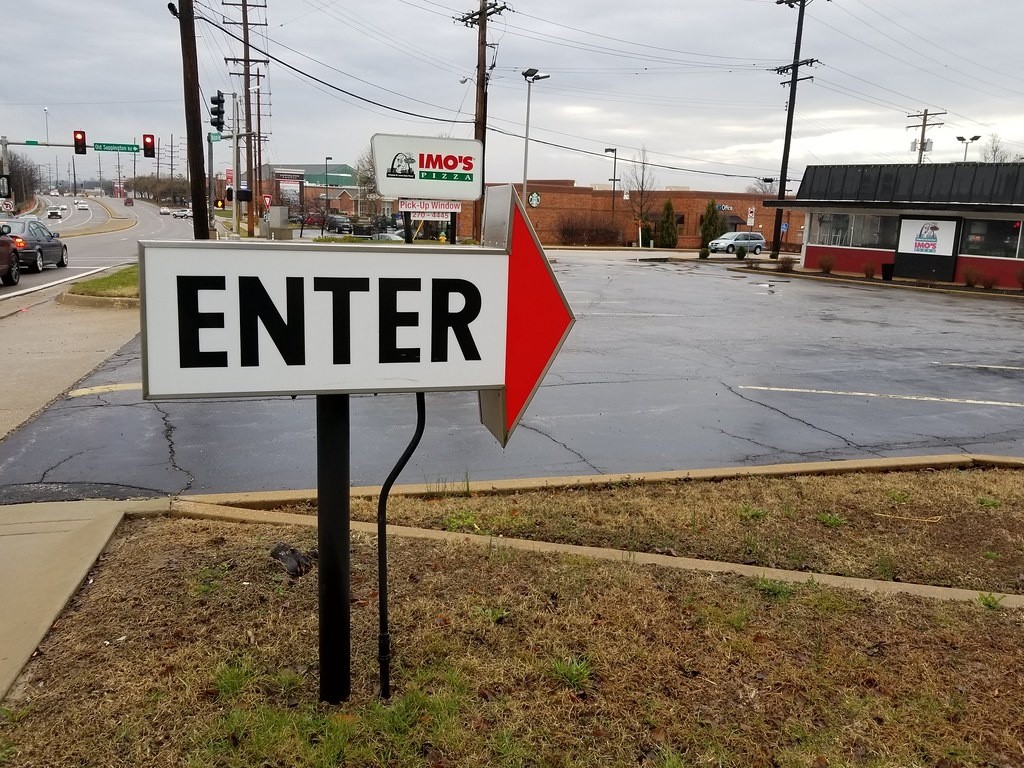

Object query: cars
[0,224,21,288]
[0,212,69,274]
[172,208,194,219]
[124,196,134,207]
[74,199,89,210]
[49,190,60,198]
[287,207,405,238]
[708,231,767,254]
[46,204,63,219]
[59,204,67,210]
[159,206,170,215]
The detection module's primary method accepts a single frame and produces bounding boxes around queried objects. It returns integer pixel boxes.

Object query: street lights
[521,67,551,211]
[459,76,489,245]
[326,156,332,216]
[605,147,617,229]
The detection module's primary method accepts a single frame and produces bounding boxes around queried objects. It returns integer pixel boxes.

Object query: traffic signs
[134,183,579,452]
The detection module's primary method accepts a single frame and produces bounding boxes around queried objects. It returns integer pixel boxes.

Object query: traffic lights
[142,134,155,158]
[74,131,87,155]
[215,199,225,209]
[210,90,226,132]
